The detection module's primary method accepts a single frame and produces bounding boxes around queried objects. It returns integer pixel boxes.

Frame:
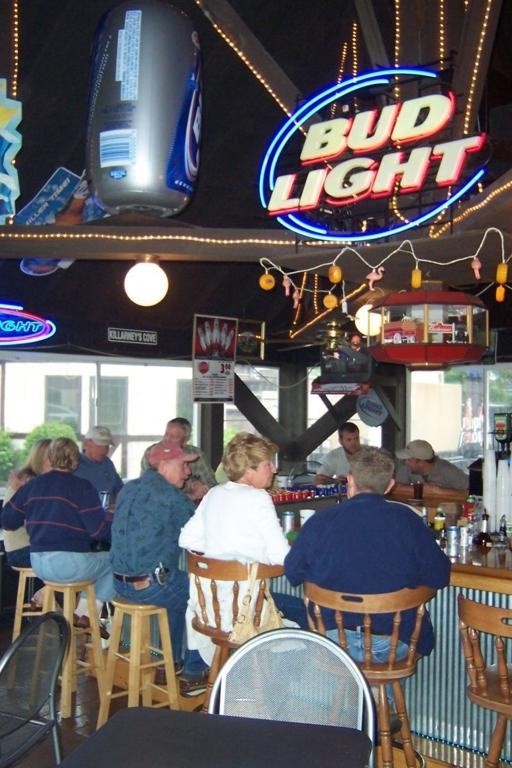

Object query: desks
[56,707,373,768]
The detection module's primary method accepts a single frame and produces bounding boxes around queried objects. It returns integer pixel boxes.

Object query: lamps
[354,303,382,336]
[123,254,169,308]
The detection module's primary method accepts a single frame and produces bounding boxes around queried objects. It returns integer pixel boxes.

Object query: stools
[8,566,47,689]
[30,581,106,721]
[96,596,180,728]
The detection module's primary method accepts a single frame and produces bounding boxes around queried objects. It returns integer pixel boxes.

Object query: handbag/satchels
[227,562,284,644]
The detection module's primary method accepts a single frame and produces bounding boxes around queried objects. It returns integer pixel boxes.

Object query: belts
[346,624,382,635]
[112,572,149,583]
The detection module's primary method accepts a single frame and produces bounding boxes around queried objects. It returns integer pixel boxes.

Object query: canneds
[446,525,458,558]
[100,490,109,510]
[281,511,296,535]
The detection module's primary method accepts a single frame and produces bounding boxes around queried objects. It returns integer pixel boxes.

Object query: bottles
[433,505,446,531]
[422,506,426,522]
[494,409,511,452]
[479,544,490,570]
[496,513,509,544]
[284,484,346,497]
[282,510,297,536]
[464,493,474,526]
[475,498,484,528]
[476,513,493,545]
[495,520,510,569]
[498,541,507,567]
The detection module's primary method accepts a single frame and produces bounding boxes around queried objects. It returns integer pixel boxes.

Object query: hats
[85,425,117,447]
[148,440,198,465]
[396,440,434,462]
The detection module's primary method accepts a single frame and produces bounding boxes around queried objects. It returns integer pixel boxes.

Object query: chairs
[207,627,376,768]
[302,580,436,768]
[458,594,512,768]
[186,550,284,714]
[0,611,68,768]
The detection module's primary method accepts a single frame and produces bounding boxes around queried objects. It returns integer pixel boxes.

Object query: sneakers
[77,615,109,639]
[73,614,80,626]
[155,659,184,684]
[31,585,62,614]
[179,672,210,698]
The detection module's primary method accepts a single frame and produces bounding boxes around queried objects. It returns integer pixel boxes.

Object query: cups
[460,527,469,544]
[482,450,496,532]
[99,489,110,509]
[496,458,512,533]
[410,482,423,500]
[299,508,314,527]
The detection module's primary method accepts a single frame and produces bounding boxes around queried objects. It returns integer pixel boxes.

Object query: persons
[2,438,64,611]
[140,417,217,497]
[311,421,371,487]
[0,438,114,639]
[394,439,469,490]
[178,432,290,668]
[284,447,452,664]
[71,425,125,507]
[109,440,211,694]
